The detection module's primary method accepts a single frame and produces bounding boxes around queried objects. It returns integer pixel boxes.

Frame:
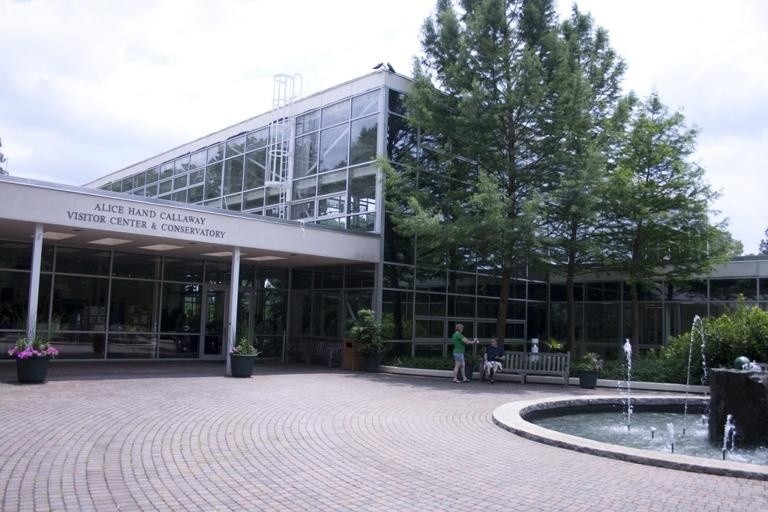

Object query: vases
[231,351,257,377]
[15,357,51,382]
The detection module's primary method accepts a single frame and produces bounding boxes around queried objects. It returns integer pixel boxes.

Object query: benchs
[478,349,572,387]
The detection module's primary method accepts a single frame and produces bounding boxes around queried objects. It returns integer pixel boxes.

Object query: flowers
[228,336,257,355]
[7,335,59,360]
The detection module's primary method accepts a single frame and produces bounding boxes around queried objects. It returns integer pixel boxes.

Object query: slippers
[452,378,471,383]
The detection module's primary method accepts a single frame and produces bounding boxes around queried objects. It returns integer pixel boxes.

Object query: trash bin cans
[342,337,356,370]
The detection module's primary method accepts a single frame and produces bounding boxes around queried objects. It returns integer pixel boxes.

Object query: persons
[483,336,506,383]
[452,323,478,383]
[175,314,185,352]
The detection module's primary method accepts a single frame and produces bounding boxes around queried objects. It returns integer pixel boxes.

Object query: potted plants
[348,307,395,371]
[578,353,602,388]
[457,348,477,382]
